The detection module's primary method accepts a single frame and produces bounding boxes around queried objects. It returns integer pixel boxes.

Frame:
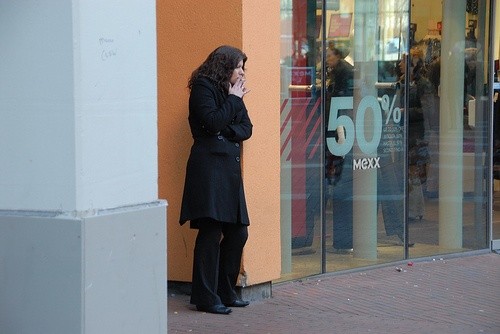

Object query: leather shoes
[194,301,232,315]
[225,299,250,307]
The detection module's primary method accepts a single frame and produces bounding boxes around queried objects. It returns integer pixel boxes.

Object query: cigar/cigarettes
[240,79,246,81]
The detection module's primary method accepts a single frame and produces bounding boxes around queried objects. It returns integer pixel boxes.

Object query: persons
[180,45,253,314]
[320,40,481,251]
